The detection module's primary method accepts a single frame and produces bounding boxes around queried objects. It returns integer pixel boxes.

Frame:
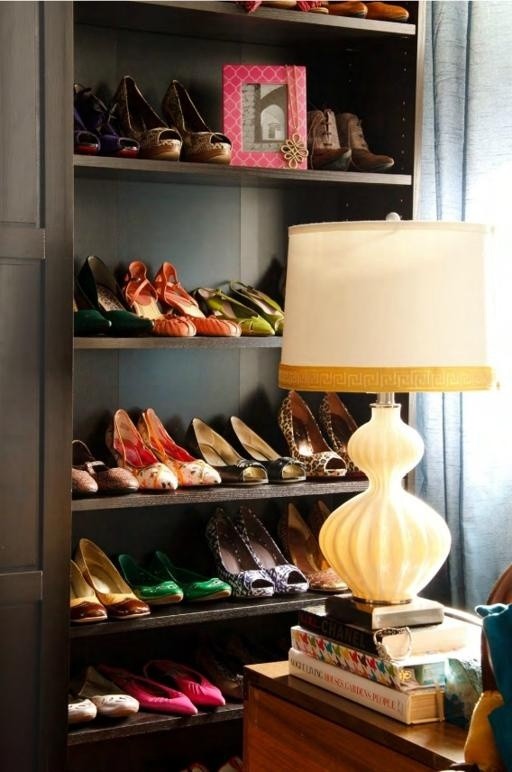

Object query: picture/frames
[220,63,309,171]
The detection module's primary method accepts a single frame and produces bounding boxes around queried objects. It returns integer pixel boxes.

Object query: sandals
[69,650,248,723]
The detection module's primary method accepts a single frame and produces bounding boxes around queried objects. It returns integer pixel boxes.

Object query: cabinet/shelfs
[67,0,421,747]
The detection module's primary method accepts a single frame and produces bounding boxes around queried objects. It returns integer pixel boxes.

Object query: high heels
[160,79,233,165]
[107,75,183,161]
[75,80,140,158]
[73,100,101,155]
[72,501,354,624]
[73,256,285,337]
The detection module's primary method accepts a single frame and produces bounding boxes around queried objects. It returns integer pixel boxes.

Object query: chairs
[478,561,512,693]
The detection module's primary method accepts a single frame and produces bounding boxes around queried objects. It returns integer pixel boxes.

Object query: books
[288,594,482,727]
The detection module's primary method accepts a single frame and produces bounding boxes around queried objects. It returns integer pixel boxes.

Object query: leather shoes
[74,393,366,496]
[248,2,413,24]
[337,112,395,173]
[307,103,354,172]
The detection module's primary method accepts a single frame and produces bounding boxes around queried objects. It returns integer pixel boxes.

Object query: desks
[238,657,485,771]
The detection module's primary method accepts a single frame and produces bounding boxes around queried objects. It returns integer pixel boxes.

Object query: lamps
[274,208,499,608]
